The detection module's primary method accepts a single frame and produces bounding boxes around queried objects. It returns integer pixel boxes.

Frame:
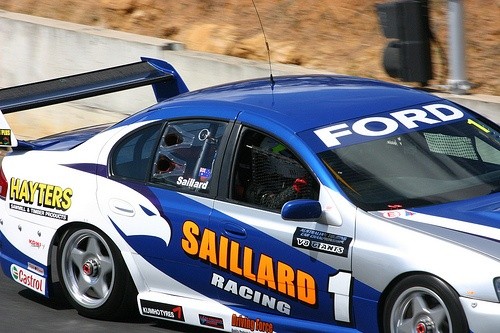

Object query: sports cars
[1,56,499,333]
